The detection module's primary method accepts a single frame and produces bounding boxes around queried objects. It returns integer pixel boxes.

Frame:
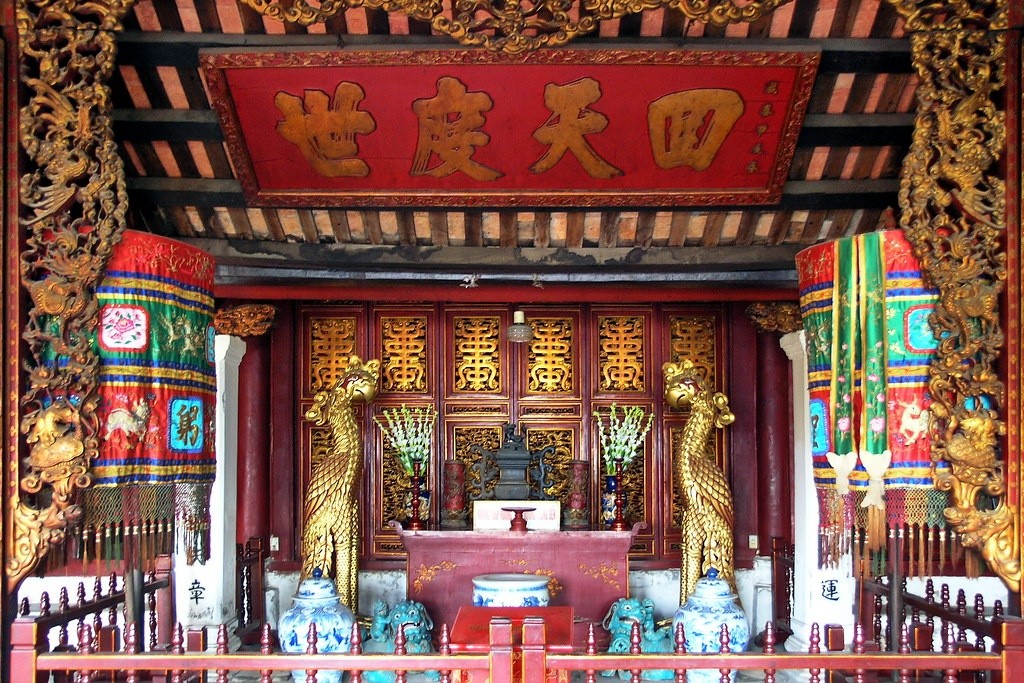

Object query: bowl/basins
[472,573,550,607]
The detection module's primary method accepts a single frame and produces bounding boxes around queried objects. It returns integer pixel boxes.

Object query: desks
[389,520,647,653]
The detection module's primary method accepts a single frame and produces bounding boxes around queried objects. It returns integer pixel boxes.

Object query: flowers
[373,403,440,478]
[586,404,654,475]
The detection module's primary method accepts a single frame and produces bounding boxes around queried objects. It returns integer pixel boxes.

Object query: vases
[603,479,628,523]
[405,474,434,528]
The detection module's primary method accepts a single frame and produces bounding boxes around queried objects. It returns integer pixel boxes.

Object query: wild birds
[303,357,381,608]
[663,357,735,597]
